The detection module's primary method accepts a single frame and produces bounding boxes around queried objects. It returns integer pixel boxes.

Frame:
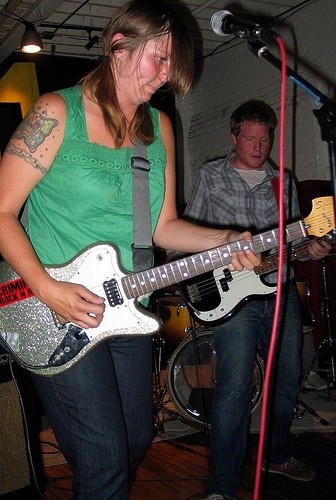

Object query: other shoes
[38,429,68,468]
[163,417,191,432]
[303,371,330,389]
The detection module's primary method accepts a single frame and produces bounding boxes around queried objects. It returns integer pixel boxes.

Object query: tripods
[299,257,336,394]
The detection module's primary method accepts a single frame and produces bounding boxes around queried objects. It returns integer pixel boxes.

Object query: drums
[166,331,266,427]
[294,278,315,334]
[154,290,205,343]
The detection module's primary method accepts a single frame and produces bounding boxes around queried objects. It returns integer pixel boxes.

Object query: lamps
[20,23,44,54]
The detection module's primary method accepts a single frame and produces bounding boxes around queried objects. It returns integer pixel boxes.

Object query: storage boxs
[156,296,205,345]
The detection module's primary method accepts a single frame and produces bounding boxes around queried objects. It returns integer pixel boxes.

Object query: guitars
[1,194,336,378]
[178,229,336,327]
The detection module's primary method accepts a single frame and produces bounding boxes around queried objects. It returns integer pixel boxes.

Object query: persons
[0,0,260,500]
[167,100,331,500]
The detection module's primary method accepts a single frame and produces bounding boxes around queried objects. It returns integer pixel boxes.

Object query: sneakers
[262,453,315,481]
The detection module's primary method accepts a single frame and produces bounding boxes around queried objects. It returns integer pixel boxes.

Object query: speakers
[0,352,47,500]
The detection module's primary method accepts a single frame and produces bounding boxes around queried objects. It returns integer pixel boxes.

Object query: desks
[290,254,336,351]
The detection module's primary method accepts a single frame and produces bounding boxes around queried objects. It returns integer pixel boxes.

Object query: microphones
[211,10,279,41]
